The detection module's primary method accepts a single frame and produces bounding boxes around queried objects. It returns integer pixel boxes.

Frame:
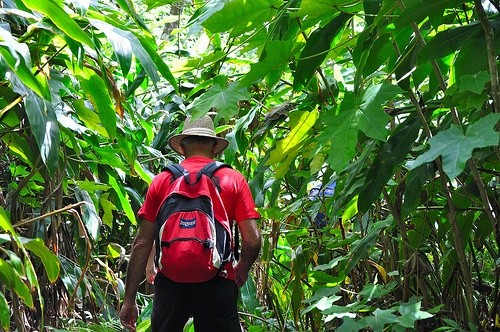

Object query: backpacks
[155,162,233,284]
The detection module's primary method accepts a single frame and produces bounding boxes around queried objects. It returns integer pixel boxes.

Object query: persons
[119,112,262,332]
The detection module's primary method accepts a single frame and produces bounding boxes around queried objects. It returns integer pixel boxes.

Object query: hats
[168,113,230,157]
